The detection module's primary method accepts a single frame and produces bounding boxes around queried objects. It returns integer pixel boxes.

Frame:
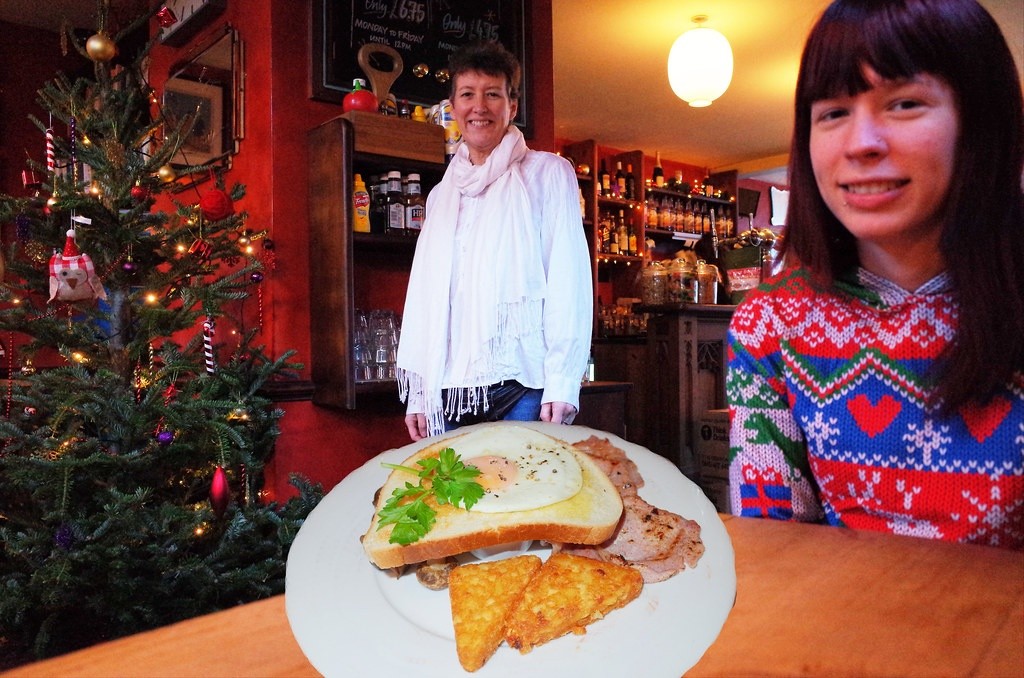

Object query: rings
[563,421,569,425]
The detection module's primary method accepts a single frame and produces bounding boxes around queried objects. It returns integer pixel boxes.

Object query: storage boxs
[694,408,732,514]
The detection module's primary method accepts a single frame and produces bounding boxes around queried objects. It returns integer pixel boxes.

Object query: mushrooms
[415,556,461,592]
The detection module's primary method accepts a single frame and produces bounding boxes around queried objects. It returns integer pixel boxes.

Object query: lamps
[667,13,734,108]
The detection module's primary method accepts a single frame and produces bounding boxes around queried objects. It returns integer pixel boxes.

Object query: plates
[285,422,737,678]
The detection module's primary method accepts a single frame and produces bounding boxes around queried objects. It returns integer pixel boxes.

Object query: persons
[725,0,1024,547]
[395,39,593,442]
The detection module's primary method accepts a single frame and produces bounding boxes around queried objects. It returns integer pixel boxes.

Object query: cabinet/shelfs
[304,109,449,411]
[560,139,740,346]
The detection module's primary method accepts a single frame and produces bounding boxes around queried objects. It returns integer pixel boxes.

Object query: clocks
[158,0,227,48]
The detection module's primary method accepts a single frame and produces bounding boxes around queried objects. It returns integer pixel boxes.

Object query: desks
[0,515,1024,678]
[631,301,738,482]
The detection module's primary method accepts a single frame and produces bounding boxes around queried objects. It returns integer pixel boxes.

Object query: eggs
[435,425,583,514]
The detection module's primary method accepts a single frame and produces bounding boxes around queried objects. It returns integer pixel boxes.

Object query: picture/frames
[162,72,231,174]
[308,0,536,142]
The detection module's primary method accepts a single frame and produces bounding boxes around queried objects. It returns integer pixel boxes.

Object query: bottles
[339,74,734,385]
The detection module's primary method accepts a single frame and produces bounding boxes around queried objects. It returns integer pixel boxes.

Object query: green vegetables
[376,447,484,544]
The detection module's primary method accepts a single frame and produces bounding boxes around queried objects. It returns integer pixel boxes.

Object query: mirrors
[150,28,234,191]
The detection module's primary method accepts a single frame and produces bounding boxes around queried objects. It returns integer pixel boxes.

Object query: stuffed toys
[47,229,107,303]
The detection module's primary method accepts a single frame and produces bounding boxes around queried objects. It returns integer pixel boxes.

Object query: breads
[361,424,624,569]
